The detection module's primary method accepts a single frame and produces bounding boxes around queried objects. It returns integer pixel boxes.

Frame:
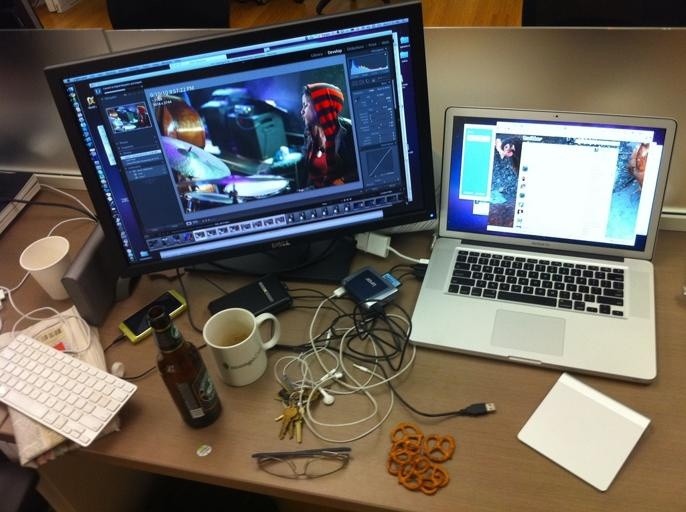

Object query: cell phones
[118,288,189,344]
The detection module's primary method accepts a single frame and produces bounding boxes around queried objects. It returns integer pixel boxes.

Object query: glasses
[252,448,351,479]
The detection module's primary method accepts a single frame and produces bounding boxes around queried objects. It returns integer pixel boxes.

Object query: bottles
[144,304,223,431]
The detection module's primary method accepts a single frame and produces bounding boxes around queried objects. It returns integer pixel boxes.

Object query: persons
[299,82,360,190]
[136,106,150,128]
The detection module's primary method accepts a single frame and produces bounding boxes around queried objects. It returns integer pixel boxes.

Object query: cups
[201,307,281,388]
[17,234,76,302]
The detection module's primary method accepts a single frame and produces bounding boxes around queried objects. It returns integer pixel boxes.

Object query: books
[0,173,41,234]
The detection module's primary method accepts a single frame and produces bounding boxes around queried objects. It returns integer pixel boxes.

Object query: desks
[0,185,684,511]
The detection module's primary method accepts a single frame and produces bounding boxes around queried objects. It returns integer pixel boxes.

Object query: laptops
[405,105,678,386]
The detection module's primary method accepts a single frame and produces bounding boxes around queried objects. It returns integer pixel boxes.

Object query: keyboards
[0,332,138,448]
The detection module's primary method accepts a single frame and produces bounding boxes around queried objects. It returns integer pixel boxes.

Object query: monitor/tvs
[41,1,439,283]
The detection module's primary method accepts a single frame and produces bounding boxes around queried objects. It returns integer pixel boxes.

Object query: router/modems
[60,217,118,328]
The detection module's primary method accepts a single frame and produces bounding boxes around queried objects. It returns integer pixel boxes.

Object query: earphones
[333,370,343,379]
[319,390,335,405]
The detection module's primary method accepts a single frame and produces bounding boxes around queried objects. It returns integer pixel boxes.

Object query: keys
[274,377,322,444]
